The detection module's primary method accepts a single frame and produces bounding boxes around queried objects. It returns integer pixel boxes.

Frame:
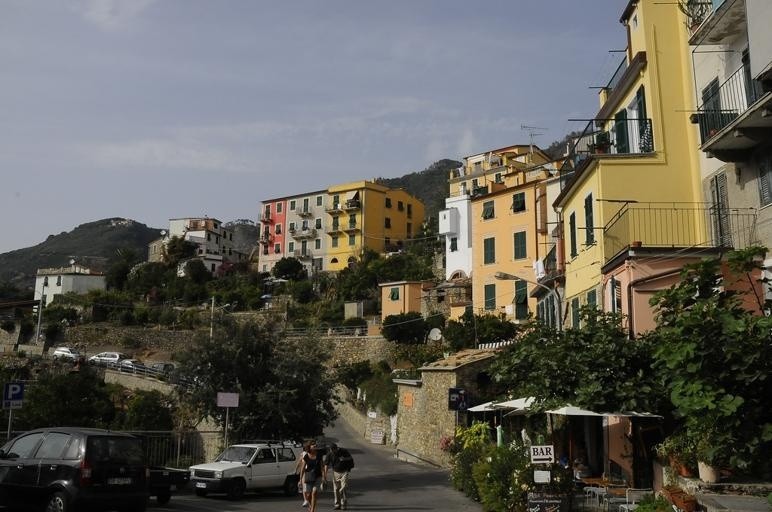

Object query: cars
[89,352,173,380]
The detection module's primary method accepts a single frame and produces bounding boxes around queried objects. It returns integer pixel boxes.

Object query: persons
[321,442,351,511]
[294,441,311,507]
[298,442,328,511]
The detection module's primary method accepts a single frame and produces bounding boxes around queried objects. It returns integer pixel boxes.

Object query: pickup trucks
[147,466,191,506]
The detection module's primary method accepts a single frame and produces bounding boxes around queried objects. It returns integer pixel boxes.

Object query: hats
[310,445,320,450]
[327,444,338,448]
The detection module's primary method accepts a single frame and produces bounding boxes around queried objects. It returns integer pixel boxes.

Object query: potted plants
[654,434,727,482]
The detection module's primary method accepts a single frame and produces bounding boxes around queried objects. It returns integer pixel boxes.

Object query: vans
[0,426,146,512]
[53,347,81,364]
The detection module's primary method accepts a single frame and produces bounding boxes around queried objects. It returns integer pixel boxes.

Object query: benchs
[584,484,654,512]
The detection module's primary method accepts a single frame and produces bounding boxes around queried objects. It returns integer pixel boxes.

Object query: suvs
[189,443,302,501]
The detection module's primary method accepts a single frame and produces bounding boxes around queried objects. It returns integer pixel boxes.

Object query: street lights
[28,286,46,345]
[211,303,231,342]
[494,271,562,328]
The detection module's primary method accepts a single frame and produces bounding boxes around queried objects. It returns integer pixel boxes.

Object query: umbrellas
[466,396,663,482]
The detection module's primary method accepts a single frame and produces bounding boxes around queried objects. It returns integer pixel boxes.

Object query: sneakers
[333,505,346,510]
[303,500,308,507]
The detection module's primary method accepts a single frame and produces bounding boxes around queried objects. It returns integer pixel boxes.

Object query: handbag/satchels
[304,470,317,483]
[343,451,354,471]
